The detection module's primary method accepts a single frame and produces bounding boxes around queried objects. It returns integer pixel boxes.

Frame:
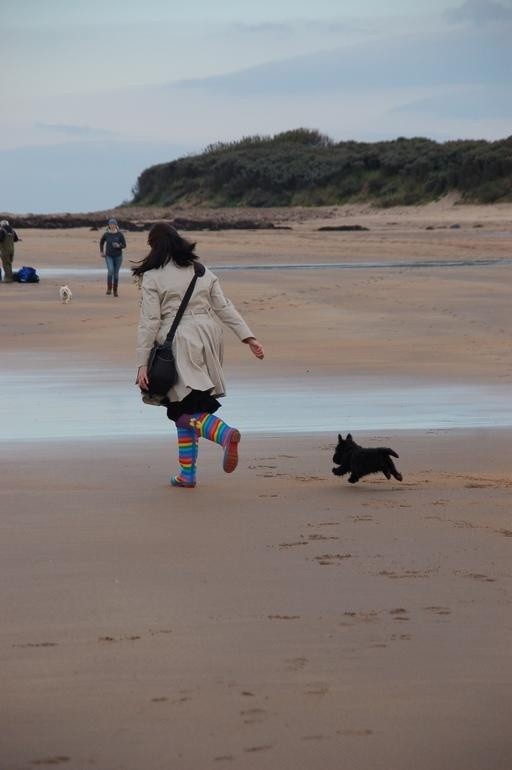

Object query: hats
[108,219,117,224]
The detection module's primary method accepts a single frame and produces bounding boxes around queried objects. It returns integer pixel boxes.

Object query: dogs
[331,433,403,484]
[59,285,72,304]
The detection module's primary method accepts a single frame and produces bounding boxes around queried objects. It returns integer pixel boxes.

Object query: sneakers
[2,278,13,283]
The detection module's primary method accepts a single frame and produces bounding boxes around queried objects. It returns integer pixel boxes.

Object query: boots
[113,284,118,296]
[171,426,198,488]
[107,282,112,294]
[192,411,240,472]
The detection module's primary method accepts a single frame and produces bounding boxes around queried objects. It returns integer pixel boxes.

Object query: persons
[100,219,126,296]
[135,221,264,488]
[0,220,18,282]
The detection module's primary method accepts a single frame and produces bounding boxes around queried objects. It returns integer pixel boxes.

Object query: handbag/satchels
[142,345,174,394]
